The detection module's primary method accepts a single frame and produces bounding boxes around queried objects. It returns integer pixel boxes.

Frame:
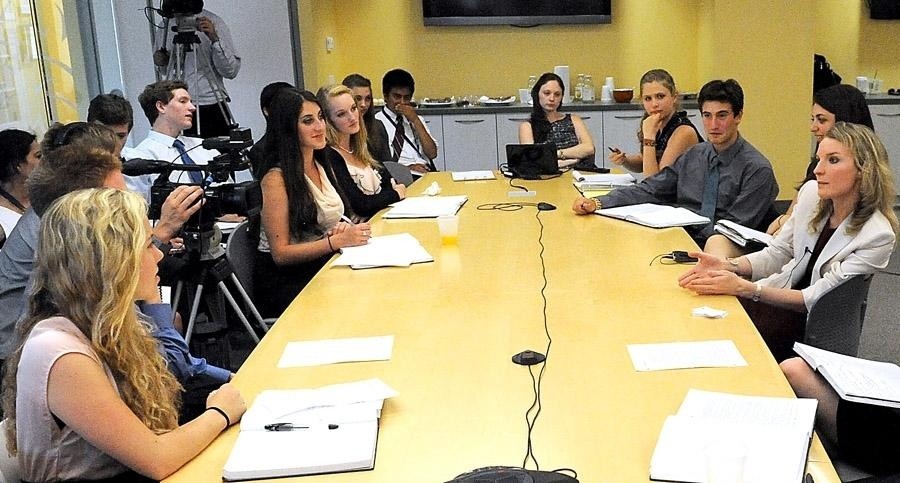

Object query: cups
[437,216,458,247]
[605,77,615,91]
[527,76,537,94]
[601,86,611,101]
[518,88,528,104]
[554,65,571,103]
[855,77,867,96]
[460,94,476,107]
[869,78,883,95]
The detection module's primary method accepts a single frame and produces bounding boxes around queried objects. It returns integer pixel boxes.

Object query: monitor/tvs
[422,0,612,27]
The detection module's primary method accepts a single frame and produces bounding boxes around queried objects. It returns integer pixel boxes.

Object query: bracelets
[590,197,602,210]
[559,148,565,160]
[643,139,656,147]
[206,407,230,427]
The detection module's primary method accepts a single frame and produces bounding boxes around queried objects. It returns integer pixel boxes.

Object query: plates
[481,95,517,105]
[420,100,455,106]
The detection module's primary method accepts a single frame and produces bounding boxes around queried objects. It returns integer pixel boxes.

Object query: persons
[136,77,249,230]
[520,72,595,168]
[572,78,779,254]
[1,186,246,483]
[609,69,705,177]
[2,91,239,427]
[677,84,898,363]
[779,358,899,481]
[151,9,241,135]
[247,69,438,319]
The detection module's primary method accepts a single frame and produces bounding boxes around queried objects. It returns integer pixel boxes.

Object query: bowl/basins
[613,88,633,104]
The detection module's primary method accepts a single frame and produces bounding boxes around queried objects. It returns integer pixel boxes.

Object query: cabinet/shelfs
[558,111,604,169]
[496,112,538,170]
[677,108,707,143]
[603,109,646,170]
[442,113,497,170]
[812,93,900,208]
[416,114,444,170]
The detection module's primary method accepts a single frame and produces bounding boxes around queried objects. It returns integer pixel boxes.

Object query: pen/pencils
[608,146,615,152]
[264,423,339,430]
[336,210,372,238]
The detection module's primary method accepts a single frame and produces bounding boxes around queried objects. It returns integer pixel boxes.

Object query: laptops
[506,143,566,175]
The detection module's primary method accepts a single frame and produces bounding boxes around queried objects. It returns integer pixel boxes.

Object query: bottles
[574,72,585,102]
[581,75,595,104]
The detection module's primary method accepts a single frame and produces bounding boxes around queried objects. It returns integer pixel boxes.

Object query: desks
[151,168,848,483]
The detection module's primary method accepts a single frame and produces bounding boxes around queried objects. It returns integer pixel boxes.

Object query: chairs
[763,197,792,236]
[755,267,876,358]
[225,215,284,326]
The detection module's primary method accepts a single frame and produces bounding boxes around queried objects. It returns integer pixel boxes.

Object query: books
[793,342,899,408]
[452,170,497,181]
[647,387,818,482]
[573,170,637,196]
[594,204,711,229]
[221,377,385,482]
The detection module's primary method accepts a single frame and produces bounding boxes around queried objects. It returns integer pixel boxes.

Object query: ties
[389,113,405,163]
[701,158,720,235]
[172,140,205,185]
